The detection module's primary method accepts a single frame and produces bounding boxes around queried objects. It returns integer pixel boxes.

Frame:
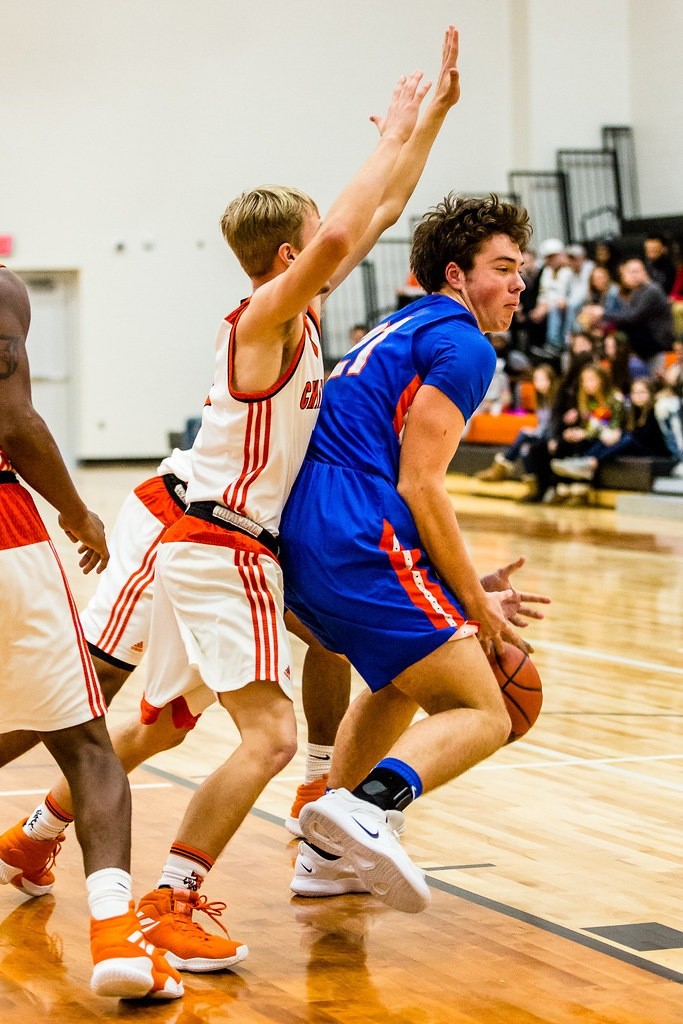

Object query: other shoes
[534,483,600,505]
[551,459,595,479]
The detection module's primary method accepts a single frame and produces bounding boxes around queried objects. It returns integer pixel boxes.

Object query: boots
[513,472,541,502]
[476,453,514,482]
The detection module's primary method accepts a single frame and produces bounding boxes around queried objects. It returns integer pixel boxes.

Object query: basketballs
[484,638,544,744]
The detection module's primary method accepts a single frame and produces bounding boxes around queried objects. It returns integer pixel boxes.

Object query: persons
[274,195,544,911]
[0,265,187,996]
[0,436,360,839]
[355,234,683,503]
[0,23,463,973]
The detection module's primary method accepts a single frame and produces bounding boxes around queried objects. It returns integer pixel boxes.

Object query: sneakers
[0,816,57,896]
[132,889,249,972]
[286,773,332,841]
[289,837,374,896]
[89,900,186,998]
[299,786,432,914]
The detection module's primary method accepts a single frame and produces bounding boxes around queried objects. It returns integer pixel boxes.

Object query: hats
[540,238,564,257]
[566,244,585,258]
